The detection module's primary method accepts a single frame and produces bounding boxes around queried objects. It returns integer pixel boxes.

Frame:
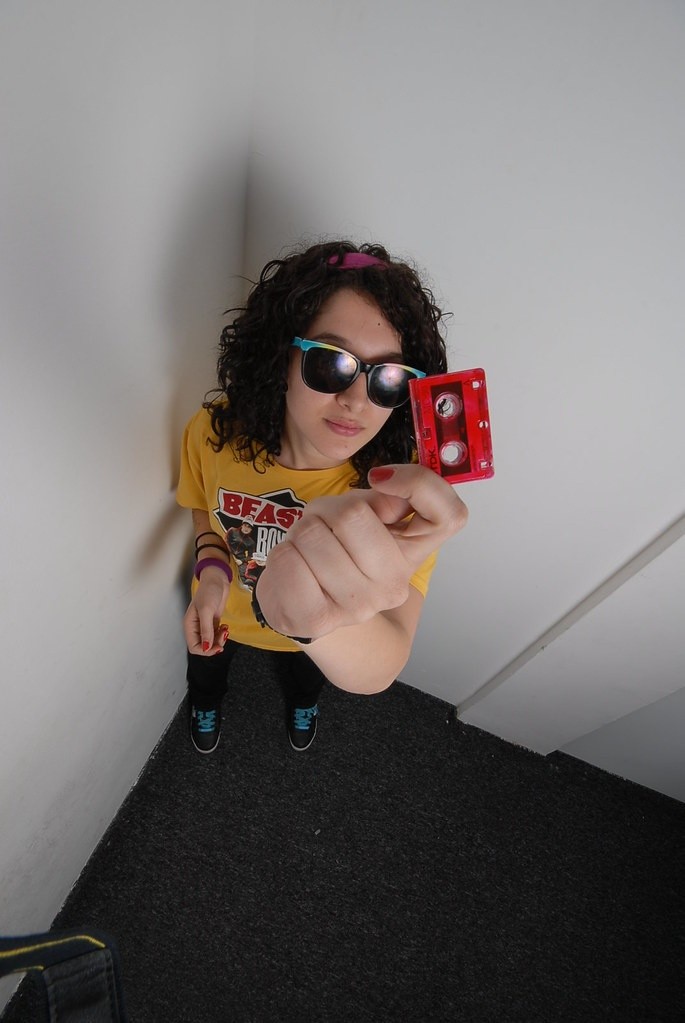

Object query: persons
[225,515,255,576]
[177,241,469,754]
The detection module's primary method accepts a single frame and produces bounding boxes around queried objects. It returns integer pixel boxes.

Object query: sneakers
[191,704,220,753]
[289,703,320,751]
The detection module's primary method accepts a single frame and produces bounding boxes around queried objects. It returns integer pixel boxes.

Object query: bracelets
[195,544,231,562]
[195,532,217,548]
[250,579,316,644]
[194,557,233,583]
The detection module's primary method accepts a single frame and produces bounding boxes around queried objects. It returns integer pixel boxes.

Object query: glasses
[282,334,426,409]
